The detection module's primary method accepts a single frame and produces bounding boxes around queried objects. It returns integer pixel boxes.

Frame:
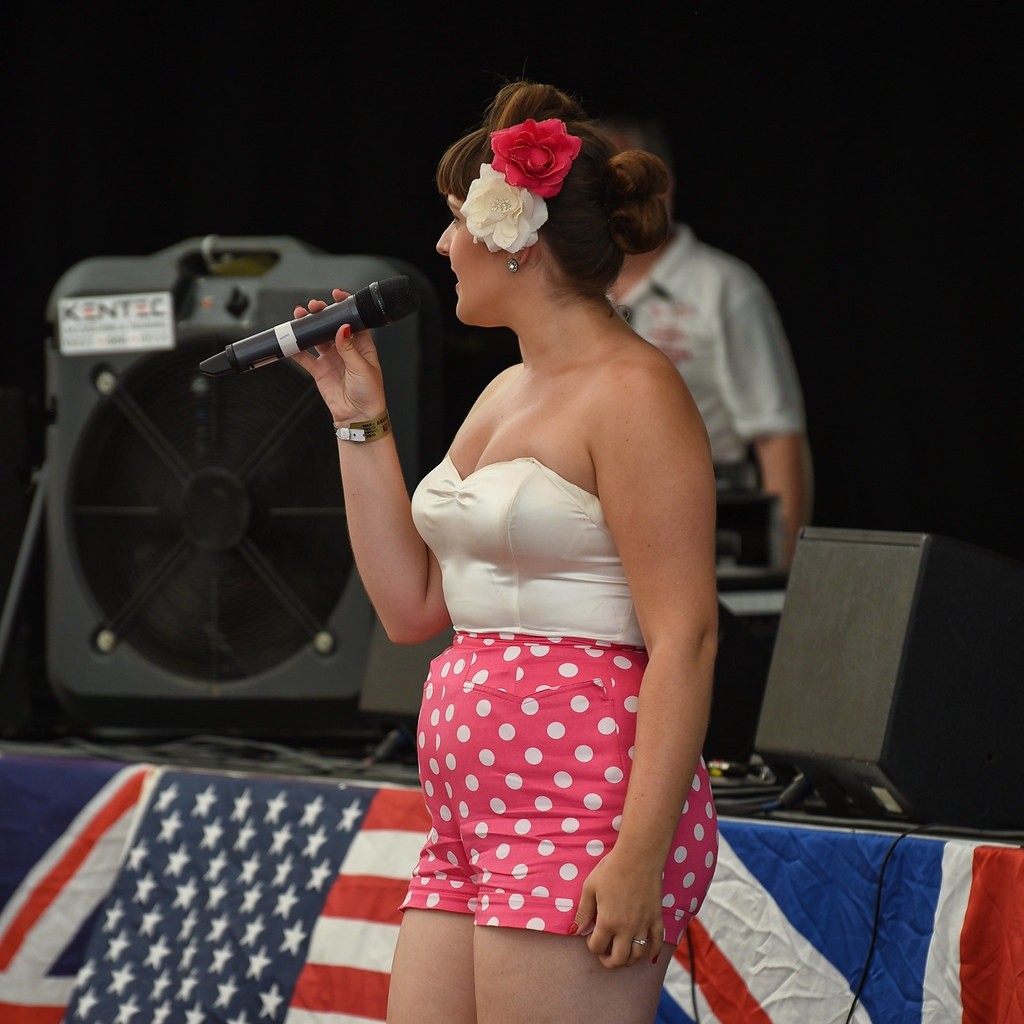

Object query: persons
[581,75,808,770]
[282,79,720,1024]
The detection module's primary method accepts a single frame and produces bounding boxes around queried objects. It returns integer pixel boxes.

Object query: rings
[632,938,651,946]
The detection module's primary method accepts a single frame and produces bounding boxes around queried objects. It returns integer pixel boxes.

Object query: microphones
[197,274,421,379]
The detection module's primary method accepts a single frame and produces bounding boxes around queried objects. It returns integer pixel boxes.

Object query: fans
[0,234,431,745]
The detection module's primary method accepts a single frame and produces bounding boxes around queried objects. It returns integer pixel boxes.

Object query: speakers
[752,526,1022,825]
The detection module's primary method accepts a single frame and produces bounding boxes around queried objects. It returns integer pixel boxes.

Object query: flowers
[459,118,584,254]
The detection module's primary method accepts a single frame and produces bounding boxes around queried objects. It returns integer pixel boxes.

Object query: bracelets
[333,410,394,444]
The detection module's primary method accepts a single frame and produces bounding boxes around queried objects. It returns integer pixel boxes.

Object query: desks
[0,740,1024,1024]
